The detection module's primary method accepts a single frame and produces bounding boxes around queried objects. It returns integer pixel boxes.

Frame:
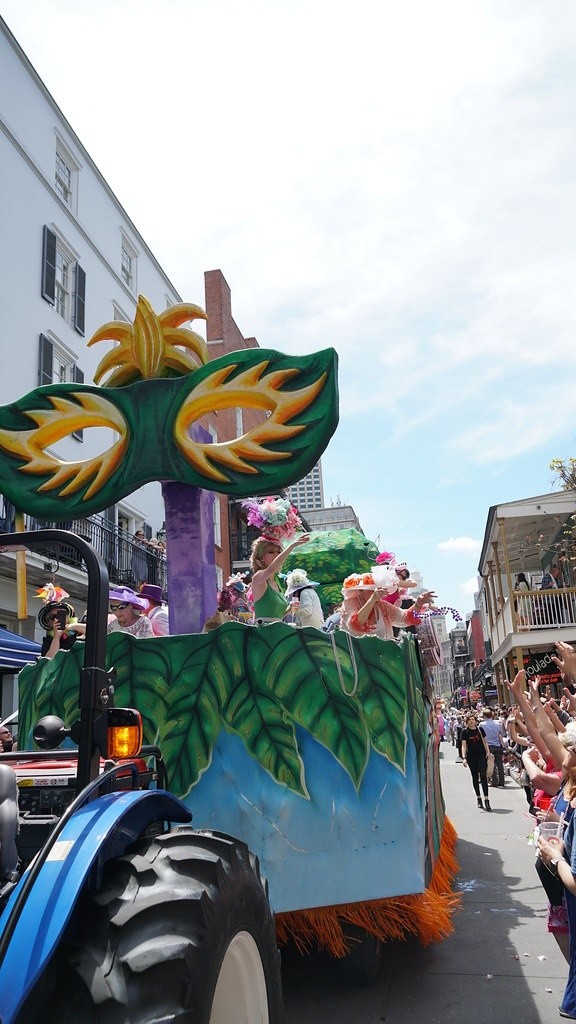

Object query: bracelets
[414,603,419,607]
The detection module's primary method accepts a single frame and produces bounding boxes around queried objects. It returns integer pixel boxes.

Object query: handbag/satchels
[486,753,496,777]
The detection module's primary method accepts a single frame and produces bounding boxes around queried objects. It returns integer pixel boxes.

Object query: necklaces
[413,607,462,622]
[358,594,377,624]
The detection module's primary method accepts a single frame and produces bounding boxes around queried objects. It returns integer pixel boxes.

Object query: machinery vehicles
[0,530,457,1024]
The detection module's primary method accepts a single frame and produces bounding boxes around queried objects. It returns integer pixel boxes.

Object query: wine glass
[291,597,298,626]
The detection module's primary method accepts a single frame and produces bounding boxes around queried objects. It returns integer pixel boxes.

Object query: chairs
[104,557,133,590]
[0,516,92,570]
[14,739,154,792]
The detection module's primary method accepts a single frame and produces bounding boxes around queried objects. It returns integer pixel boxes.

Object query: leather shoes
[485,799,492,810]
[477,798,483,808]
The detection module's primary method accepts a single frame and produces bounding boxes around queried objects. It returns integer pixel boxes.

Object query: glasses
[109,602,129,611]
[0,731,11,735]
[399,573,407,580]
[46,613,57,623]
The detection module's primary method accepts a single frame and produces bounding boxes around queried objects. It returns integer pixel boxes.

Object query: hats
[283,568,321,597]
[32,583,76,630]
[108,585,150,610]
[136,584,167,603]
[340,566,399,598]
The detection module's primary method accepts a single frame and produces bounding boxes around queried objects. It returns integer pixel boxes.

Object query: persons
[0,726,18,753]
[496,568,569,632]
[38,498,445,658]
[444,641,576,1018]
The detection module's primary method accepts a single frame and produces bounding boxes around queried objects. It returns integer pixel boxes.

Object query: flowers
[342,575,375,587]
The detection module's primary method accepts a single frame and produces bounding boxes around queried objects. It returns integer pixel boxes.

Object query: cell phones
[57,609,67,632]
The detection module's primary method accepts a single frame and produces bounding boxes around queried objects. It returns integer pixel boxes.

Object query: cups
[538,798,551,809]
[538,822,564,856]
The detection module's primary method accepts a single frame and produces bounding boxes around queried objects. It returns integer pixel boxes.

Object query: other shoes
[488,783,496,787]
[557,1007,576,1018]
